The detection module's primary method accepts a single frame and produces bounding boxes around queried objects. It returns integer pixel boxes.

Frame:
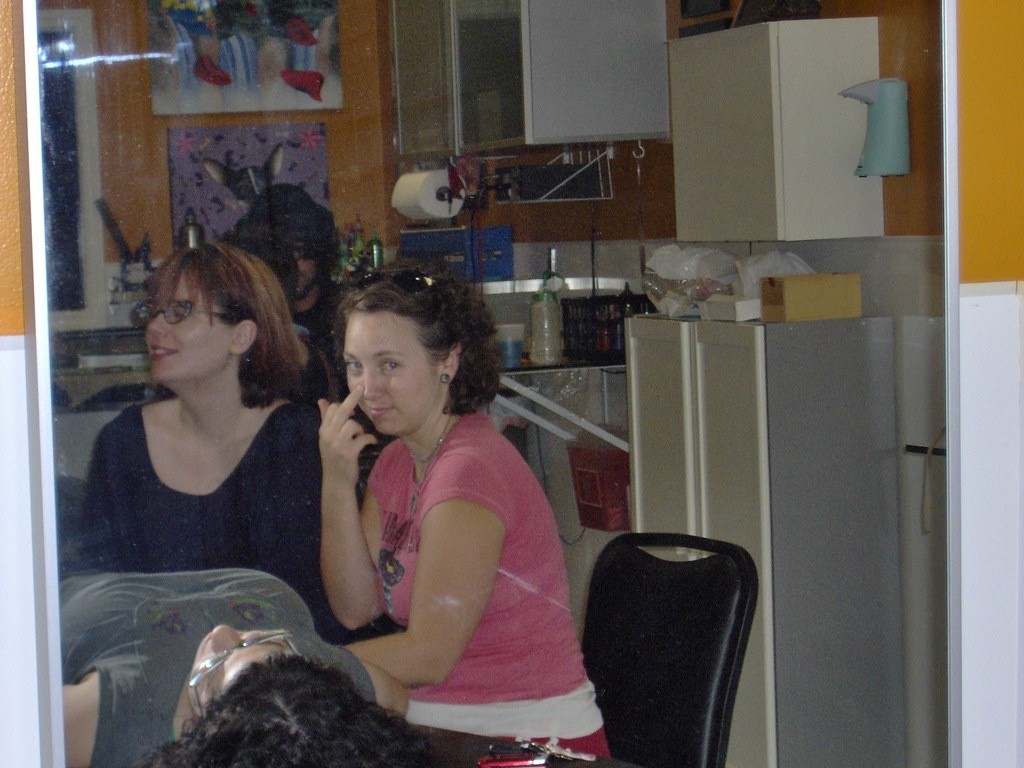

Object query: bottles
[178,208,203,249]
[530,291,563,366]
[334,217,384,268]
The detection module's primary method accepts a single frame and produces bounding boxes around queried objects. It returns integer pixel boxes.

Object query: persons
[59,565,443,768]
[220,185,338,360]
[76,237,401,647]
[318,257,609,768]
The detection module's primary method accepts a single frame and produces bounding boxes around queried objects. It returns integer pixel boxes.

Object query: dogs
[223,181,337,406]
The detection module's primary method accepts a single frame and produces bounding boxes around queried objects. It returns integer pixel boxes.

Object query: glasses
[362,271,439,294]
[187,633,302,720]
[135,297,239,325]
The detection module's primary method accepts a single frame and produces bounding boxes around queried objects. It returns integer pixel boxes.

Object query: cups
[494,324,525,369]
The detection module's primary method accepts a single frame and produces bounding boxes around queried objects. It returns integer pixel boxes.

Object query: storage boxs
[760,270,863,325]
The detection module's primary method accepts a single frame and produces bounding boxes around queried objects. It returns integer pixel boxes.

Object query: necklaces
[406,412,452,462]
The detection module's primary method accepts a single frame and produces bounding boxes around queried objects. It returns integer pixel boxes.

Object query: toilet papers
[390,167,467,221]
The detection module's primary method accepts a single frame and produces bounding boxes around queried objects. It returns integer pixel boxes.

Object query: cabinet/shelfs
[669,18,884,240]
[624,314,908,768]
[390,0,668,160]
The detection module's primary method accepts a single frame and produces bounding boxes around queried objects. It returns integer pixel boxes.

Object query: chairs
[581,533,759,768]
